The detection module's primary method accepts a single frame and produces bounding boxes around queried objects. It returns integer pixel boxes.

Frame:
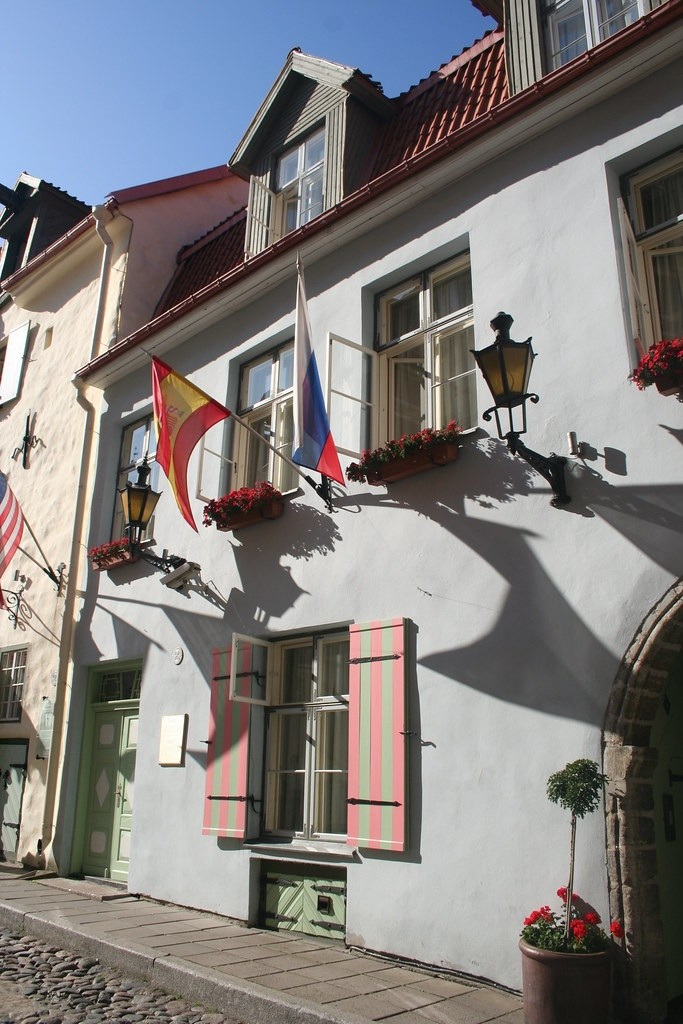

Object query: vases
[91,548,140,571]
[217,501,284,532]
[519,929,611,1024]
[654,372,683,397]
[366,443,457,485]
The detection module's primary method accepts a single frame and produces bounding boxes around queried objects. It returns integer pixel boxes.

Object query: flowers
[516,750,633,950]
[87,537,136,560]
[626,335,683,392]
[342,424,474,484]
[203,483,285,532]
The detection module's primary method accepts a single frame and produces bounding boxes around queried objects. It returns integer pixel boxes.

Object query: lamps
[159,562,208,594]
[470,310,571,507]
[116,450,187,590]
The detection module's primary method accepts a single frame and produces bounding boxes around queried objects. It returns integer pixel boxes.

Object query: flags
[290,263,348,490]
[0,470,25,574]
[150,356,228,536]
